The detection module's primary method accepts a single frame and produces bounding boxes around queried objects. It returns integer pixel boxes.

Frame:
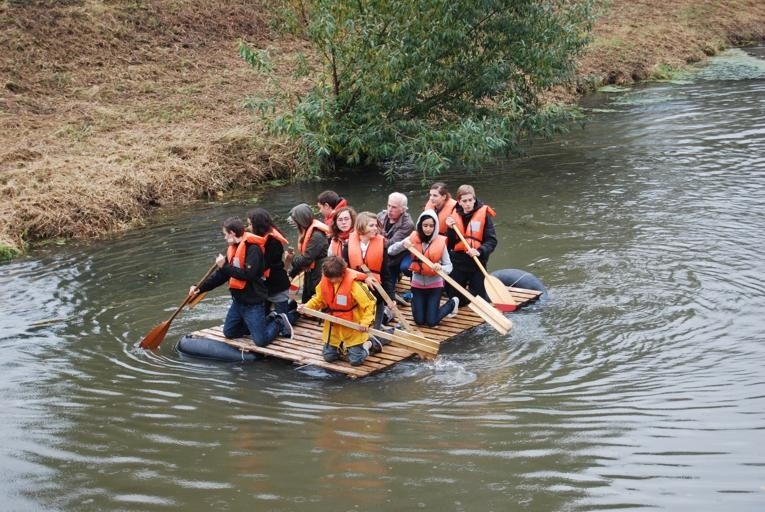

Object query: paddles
[139,260,214,349]
[289,272,305,291]
[452,223,516,304]
[408,245,513,335]
[360,264,440,361]
[301,307,441,358]
[187,289,207,311]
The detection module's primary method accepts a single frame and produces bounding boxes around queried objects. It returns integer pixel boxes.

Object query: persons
[445,185,498,307]
[246,208,303,325]
[316,190,347,245]
[341,211,403,345]
[295,255,384,367]
[326,206,357,270]
[424,181,457,235]
[377,192,415,281]
[188,215,293,347]
[387,209,460,326]
[284,204,329,320]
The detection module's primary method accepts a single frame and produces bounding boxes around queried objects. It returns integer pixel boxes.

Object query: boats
[171,260,551,378]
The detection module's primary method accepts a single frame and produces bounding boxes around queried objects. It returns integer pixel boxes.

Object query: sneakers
[446,296,459,318]
[400,292,413,302]
[384,305,394,322]
[274,313,293,340]
[368,335,383,355]
